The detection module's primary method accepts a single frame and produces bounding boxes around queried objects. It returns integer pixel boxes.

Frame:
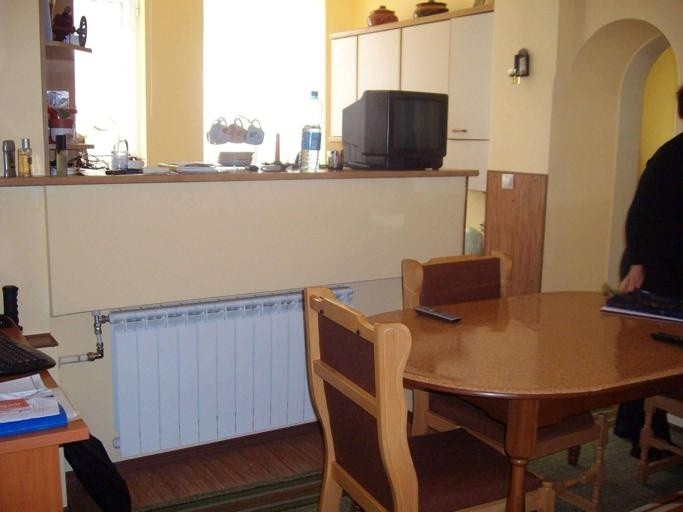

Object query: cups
[55,90,70,109]
[47,90,56,107]
[110,139,129,172]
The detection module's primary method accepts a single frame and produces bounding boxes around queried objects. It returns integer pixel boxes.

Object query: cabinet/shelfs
[0,315,88,512]
[401,5,494,141]
[44,40,93,167]
[331,21,401,138]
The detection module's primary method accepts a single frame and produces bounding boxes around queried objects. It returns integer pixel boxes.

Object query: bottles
[2,133,68,179]
[298,90,324,175]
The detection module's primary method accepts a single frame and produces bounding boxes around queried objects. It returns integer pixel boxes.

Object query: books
[0,373,68,439]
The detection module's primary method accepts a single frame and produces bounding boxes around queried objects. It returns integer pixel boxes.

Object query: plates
[216,152,255,168]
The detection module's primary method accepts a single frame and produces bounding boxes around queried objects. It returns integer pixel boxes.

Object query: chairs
[400,253,608,512]
[303,286,556,512]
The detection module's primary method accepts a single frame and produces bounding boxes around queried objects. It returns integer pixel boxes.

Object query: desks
[365,291,683,511]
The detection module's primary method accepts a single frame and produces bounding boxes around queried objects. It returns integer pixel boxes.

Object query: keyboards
[0,330,56,377]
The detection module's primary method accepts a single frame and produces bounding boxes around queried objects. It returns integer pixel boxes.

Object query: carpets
[134,467,354,511]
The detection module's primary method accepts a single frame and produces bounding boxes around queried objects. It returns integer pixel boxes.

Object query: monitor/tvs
[343,90,448,170]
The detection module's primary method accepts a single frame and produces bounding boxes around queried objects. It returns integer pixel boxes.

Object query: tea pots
[206,117,264,146]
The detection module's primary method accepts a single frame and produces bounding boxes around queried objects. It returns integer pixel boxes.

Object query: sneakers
[630,441,679,462]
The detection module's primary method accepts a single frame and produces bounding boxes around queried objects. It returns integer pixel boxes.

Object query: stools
[625,488,683,512]
[635,390,683,485]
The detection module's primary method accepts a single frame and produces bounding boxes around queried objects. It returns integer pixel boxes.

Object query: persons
[614,86,683,461]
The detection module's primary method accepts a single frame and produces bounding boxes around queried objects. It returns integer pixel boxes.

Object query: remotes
[414,306,463,323]
[650,332,683,345]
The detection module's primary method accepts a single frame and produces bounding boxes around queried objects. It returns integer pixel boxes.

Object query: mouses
[0,318,13,328]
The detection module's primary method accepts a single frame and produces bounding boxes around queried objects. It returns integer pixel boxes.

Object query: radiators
[59,286,355,457]
